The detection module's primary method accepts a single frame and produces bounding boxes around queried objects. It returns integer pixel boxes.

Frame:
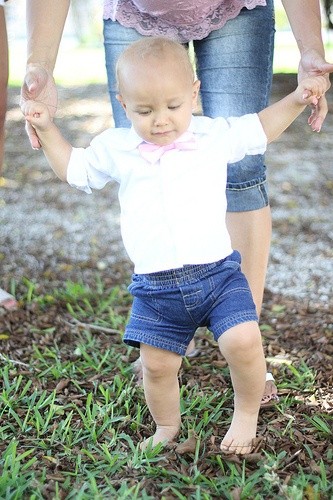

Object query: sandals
[259,372,281,410]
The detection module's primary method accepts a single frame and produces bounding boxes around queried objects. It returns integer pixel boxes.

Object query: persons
[0,0,18,313]
[21,35,328,456]
[19,0,333,407]
